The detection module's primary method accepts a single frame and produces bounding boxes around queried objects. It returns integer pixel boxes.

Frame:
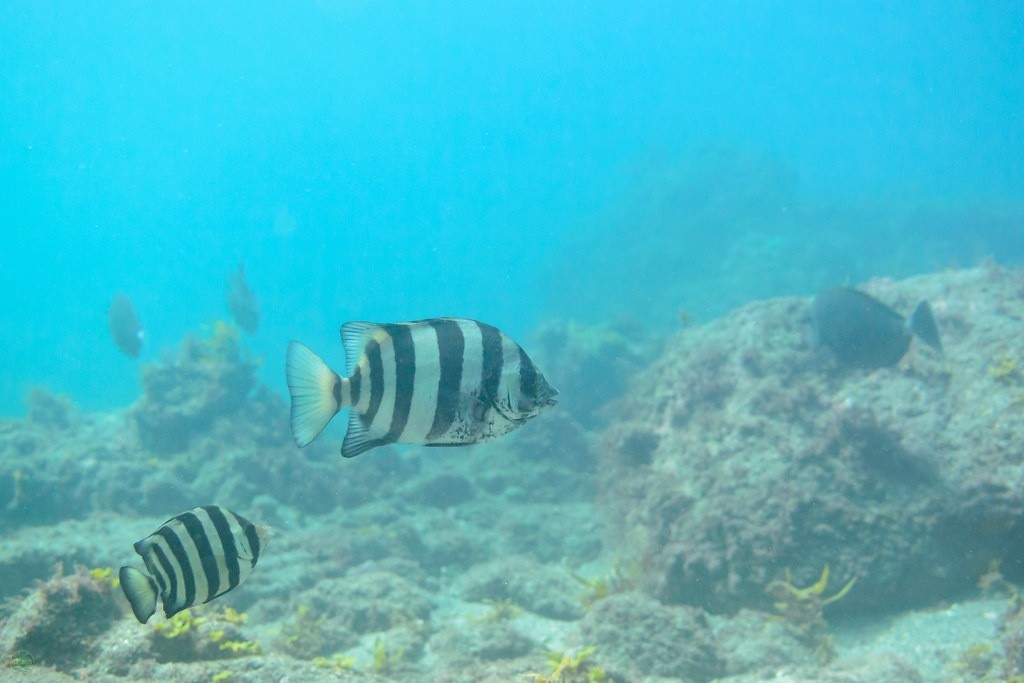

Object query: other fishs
[811,288,943,368]
[108,297,144,357]
[227,272,258,335]
[285,318,559,458]
[119,504,272,624]
